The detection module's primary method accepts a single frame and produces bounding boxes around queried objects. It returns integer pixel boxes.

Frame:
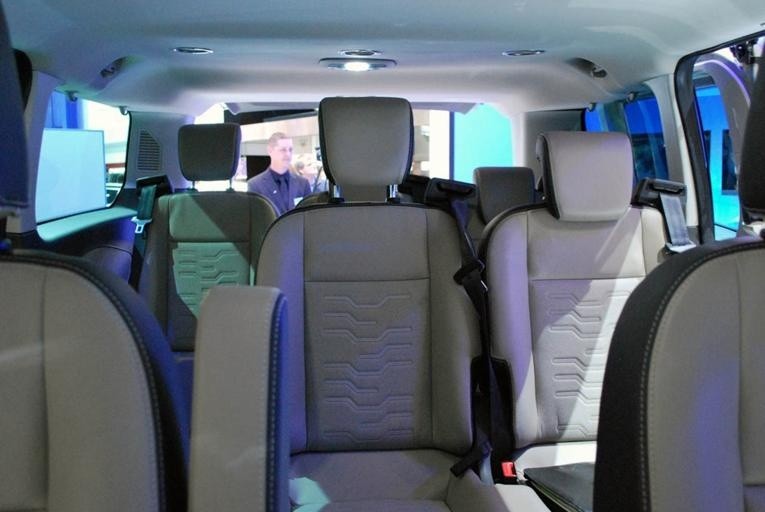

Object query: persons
[294,152,331,196]
[244,131,313,221]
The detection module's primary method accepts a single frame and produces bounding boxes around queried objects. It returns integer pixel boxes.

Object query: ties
[278,175,289,210]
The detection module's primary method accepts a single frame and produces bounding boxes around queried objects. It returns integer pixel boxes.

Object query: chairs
[3,80,184,512]
[476,131,691,510]
[592,79,764,512]
[139,125,283,368]
[188,96,551,510]
